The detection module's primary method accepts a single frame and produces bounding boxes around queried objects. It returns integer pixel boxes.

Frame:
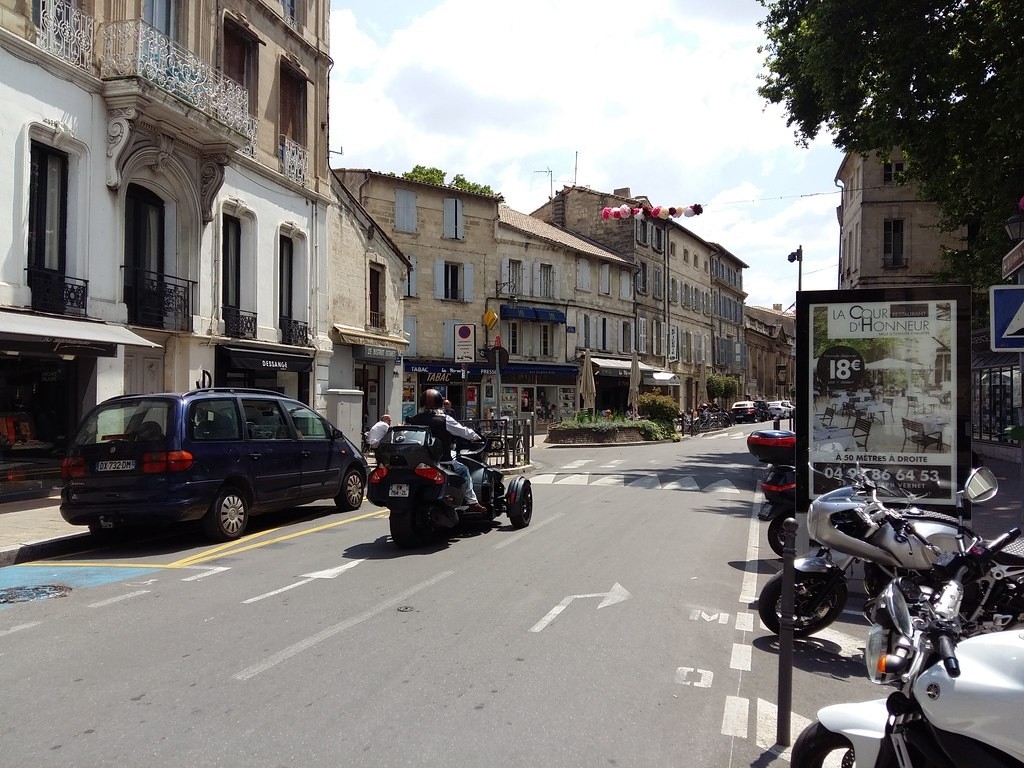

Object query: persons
[404,415,413,425]
[367,415,391,448]
[605,410,613,421]
[443,400,456,420]
[411,389,487,512]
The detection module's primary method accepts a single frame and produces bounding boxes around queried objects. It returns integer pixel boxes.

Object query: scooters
[747,429,797,558]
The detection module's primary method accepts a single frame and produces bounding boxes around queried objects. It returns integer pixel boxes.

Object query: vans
[59,387,372,542]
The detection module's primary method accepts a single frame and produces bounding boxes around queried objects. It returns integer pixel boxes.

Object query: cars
[755,400,774,422]
[731,402,766,423]
[767,401,795,420]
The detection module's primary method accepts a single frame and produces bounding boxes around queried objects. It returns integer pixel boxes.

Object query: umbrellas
[580,349,596,416]
[865,358,927,370]
[628,350,641,422]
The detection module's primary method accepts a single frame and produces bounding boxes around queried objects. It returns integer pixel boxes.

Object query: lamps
[494,279,519,311]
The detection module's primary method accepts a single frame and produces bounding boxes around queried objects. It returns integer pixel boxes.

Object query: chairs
[135,420,162,440]
[194,415,235,439]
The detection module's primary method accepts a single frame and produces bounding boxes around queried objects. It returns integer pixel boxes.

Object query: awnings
[589,357,654,378]
[0,311,163,348]
[500,305,536,321]
[333,323,410,351]
[532,308,566,324]
[643,373,680,385]
[405,362,580,376]
[220,346,314,372]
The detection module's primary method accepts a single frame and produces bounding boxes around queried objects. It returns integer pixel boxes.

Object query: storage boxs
[364,467,390,506]
[373,425,442,468]
[411,458,466,508]
[747,429,794,466]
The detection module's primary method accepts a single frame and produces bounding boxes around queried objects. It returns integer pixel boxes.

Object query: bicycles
[675,408,728,435]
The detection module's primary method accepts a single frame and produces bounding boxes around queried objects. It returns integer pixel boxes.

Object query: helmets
[419,389,444,409]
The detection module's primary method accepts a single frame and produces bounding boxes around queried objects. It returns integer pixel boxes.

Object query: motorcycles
[756,461,1024,640]
[787,467,1024,768]
[366,426,533,550]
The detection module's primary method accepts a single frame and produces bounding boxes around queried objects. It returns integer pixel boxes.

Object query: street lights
[485,294,518,352]
[787,245,803,291]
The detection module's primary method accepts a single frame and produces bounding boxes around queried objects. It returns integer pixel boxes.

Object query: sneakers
[465,504,487,512]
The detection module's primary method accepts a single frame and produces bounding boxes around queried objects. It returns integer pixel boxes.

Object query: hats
[605,409,610,413]
